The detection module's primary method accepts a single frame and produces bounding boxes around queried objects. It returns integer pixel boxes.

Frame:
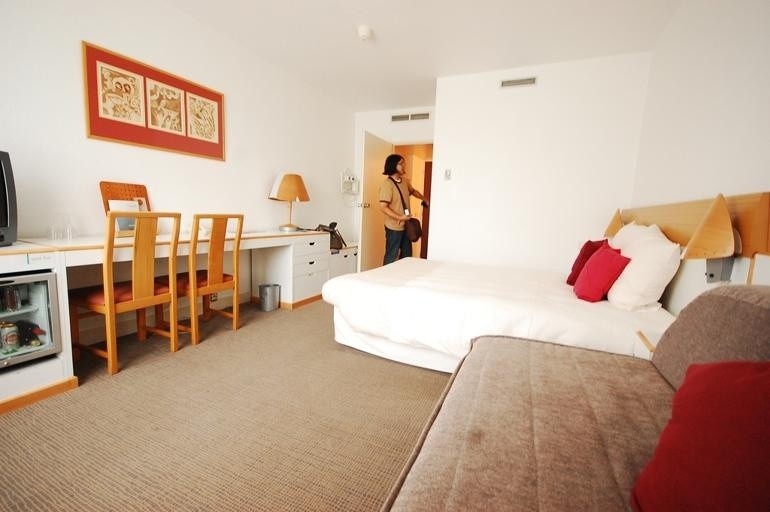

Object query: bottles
[15,319,45,346]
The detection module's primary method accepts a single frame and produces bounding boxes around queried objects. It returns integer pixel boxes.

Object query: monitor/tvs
[0,151,17,246]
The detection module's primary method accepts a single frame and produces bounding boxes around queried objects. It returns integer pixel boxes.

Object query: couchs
[376,283,770,512]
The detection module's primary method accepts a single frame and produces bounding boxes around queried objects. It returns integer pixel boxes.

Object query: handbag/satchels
[315,222,348,250]
[405,218,423,243]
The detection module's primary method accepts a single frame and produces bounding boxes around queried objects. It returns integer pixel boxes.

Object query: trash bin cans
[258,284,281,312]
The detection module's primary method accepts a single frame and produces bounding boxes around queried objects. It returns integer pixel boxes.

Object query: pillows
[609,220,646,254]
[629,358,770,511]
[606,224,681,311]
[573,241,630,302]
[566,238,607,286]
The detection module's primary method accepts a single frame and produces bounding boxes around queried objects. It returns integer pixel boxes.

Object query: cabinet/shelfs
[330,245,358,278]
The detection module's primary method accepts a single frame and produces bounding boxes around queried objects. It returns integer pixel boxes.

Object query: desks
[0,230,331,415]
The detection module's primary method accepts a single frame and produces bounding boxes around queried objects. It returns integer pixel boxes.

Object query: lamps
[269,173,310,231]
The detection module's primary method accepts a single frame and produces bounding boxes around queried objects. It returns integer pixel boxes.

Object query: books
[108,199,139,231]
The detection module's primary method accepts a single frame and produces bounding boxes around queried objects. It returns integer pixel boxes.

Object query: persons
[379,154,429,266]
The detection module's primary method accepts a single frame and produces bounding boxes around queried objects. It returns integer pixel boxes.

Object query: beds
[321,191,770,375]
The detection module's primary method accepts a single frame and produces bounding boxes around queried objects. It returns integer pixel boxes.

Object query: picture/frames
[80,40,226,163]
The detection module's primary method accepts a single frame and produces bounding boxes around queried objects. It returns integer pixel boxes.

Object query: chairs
[69,211,181,374]
[155,213,244,346]
